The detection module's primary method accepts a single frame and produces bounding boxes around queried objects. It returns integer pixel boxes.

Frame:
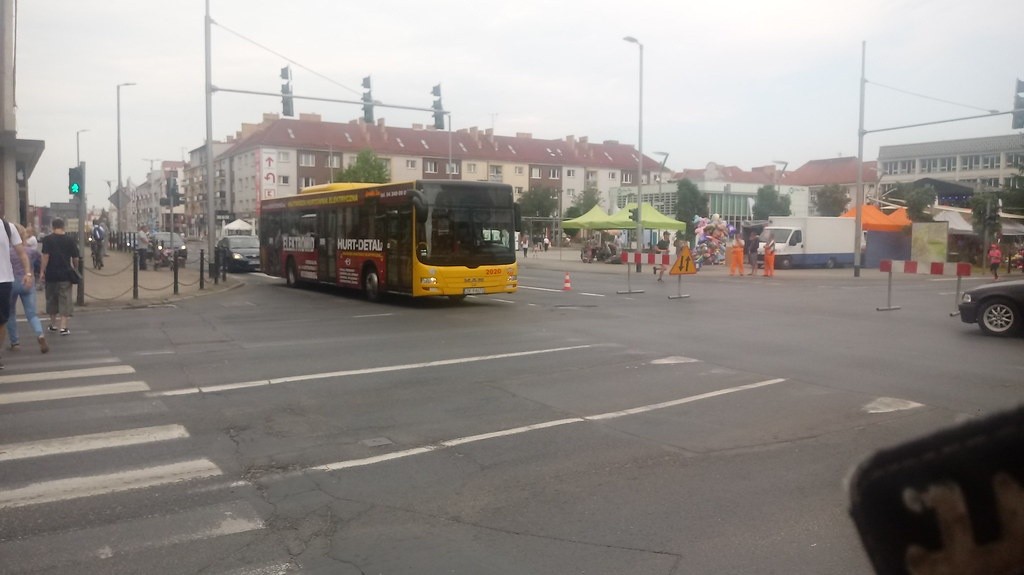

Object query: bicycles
[89,240,103,270]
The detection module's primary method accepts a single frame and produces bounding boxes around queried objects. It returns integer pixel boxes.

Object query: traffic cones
[562,272,572,291]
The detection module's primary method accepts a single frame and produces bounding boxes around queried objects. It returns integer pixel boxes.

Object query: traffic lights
[1010,77,1024,130]
[628,207,638,223]
[68,169,81,194]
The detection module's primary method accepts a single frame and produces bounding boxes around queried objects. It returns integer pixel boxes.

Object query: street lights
[117,81,138,244]
[142,157,161,232]
[76,128,89,167]
[623,36,643,272]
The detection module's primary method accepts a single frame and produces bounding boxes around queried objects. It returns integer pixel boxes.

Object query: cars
[957,280,1024,337]
[122,233,138,251]
[152,231,188,259]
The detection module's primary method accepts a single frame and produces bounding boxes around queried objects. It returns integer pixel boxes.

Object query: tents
[887,206,913,227]
[839,203,903,233]
[583,199,686,250]
[561,204,618,238]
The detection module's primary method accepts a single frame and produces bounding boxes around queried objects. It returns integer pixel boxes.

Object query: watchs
[23,272,32,277]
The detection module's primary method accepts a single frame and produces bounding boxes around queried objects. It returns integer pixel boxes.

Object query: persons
[138,226,152,270]
[730,232,745,276]
[520,236,529,257]
[761,233,775,277]
[585,236,598,263]
[40,216,80,336]
[653,231,671,282]
[543,236,549,251]
[562,231,571,246]
[533,234,537,246]
[601,243,612,258]
[0,217,49,370]
[988,244,1001,281]
[89,219,106,267]
[537,234,543,250]
[745,229,760,275]
[1016,249,1024,273]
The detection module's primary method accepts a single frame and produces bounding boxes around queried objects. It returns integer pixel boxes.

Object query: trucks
[748,217,863,270]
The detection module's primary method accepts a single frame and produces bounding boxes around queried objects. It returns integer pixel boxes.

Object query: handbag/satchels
[67,237,83,284]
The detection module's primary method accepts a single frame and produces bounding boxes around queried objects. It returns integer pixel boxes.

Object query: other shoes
[657,279,664,283]
[653,266,656,274]
[48,325,58,332]
[38,336,49,352]
[12,344,19,349]
[60,328,70,335]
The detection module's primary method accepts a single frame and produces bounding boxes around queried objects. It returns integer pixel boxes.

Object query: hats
[992,244,996,248]
[663,231,671,236]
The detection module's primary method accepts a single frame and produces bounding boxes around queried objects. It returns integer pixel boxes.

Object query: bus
[217,236,263,274]
[259,178,523,304]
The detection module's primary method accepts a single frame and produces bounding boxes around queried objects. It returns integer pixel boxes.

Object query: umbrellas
[223,218,252,235]
